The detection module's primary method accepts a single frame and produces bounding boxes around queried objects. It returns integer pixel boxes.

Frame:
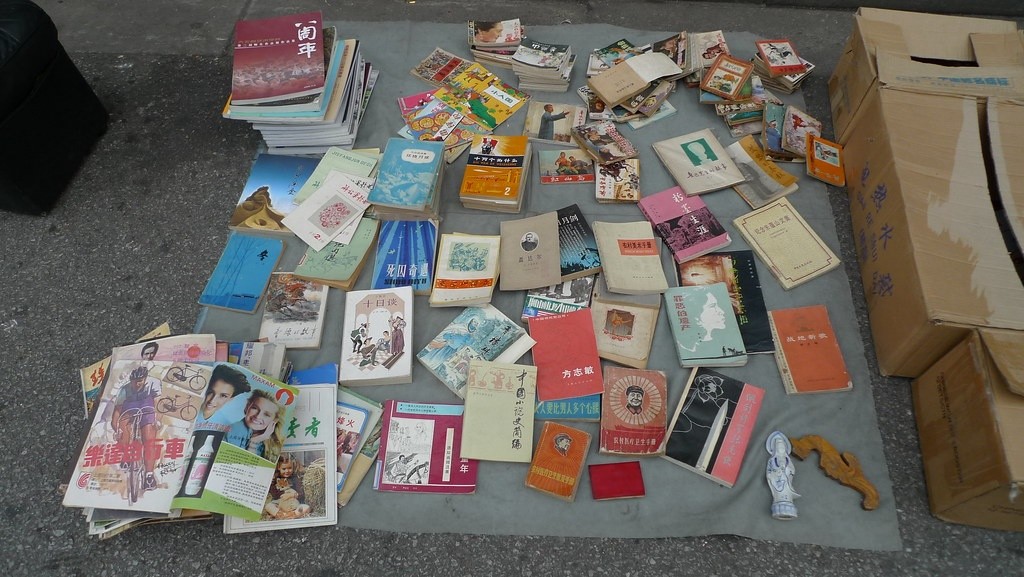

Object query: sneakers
[121,451,131,470]
[144,471,156,490]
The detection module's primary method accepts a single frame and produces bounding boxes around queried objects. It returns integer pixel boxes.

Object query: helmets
[129,366,148,381]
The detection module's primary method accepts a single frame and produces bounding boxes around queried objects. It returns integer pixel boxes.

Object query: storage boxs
[842,47,1024,378]
[827,6,1024,146]
[908,328,1024,532]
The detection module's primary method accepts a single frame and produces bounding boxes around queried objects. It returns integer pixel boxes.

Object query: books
[63,10,854,540]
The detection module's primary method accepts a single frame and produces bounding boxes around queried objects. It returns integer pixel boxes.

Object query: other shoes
[274,511,296,519]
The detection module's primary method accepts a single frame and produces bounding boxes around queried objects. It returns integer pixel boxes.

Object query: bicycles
[157,394,198,421]
[112,405,158,503]
[166,364,207,391]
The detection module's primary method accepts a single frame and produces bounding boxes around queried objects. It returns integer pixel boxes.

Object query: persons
[195,365,252,431]
[140,342,159,361]
[264,453,310,519]
[224,389,278,457]
[474,20,503,44]
[110,368,161,492]
[538,104,571,140]
[766,430,802,520]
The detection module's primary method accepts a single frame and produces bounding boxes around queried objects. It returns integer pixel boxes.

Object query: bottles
[174,434,196,496]
[183,435,215,495]
